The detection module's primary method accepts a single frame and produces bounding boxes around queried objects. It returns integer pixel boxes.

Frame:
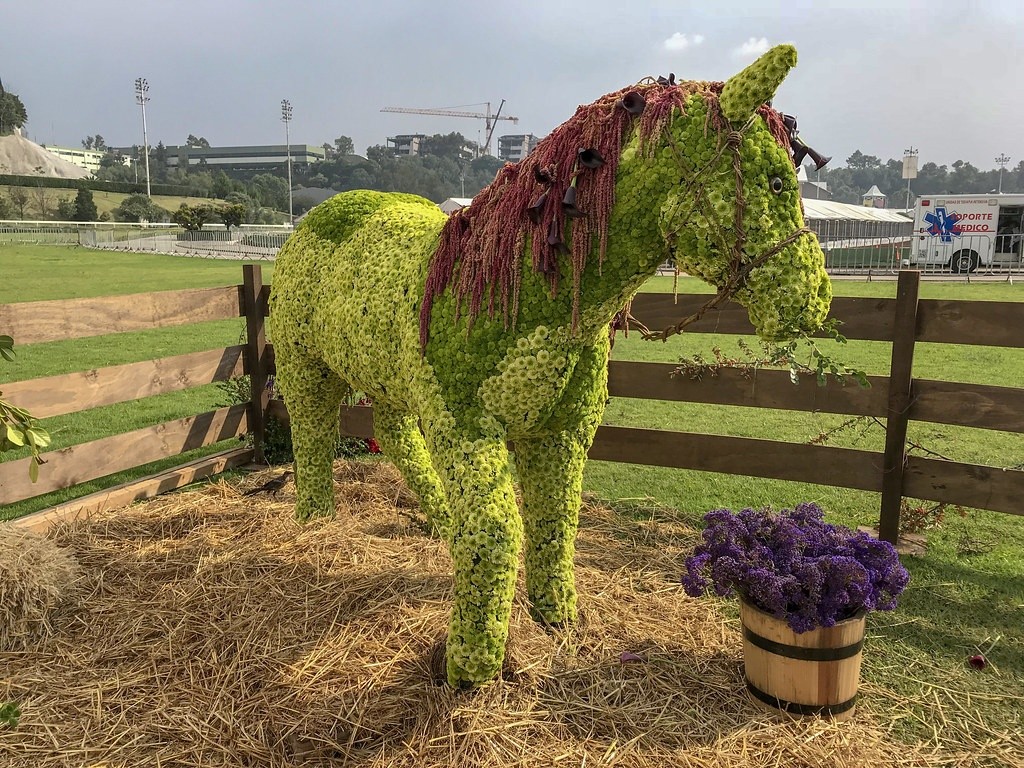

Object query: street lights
[135,78,151,199]
[280,99,296,226]
[995,151,1014,194]
[903,145,919,213]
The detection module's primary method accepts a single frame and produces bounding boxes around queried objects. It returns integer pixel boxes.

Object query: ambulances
[908,193,1024,273]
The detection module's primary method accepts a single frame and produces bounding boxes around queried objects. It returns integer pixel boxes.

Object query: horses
[268,44,832,689]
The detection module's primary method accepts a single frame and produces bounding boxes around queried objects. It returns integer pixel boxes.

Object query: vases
[736,590,868,725]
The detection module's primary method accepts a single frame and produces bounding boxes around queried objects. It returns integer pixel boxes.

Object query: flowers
[678,499,911,635]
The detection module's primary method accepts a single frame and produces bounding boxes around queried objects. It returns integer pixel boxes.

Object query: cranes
[379,98,520,158]
